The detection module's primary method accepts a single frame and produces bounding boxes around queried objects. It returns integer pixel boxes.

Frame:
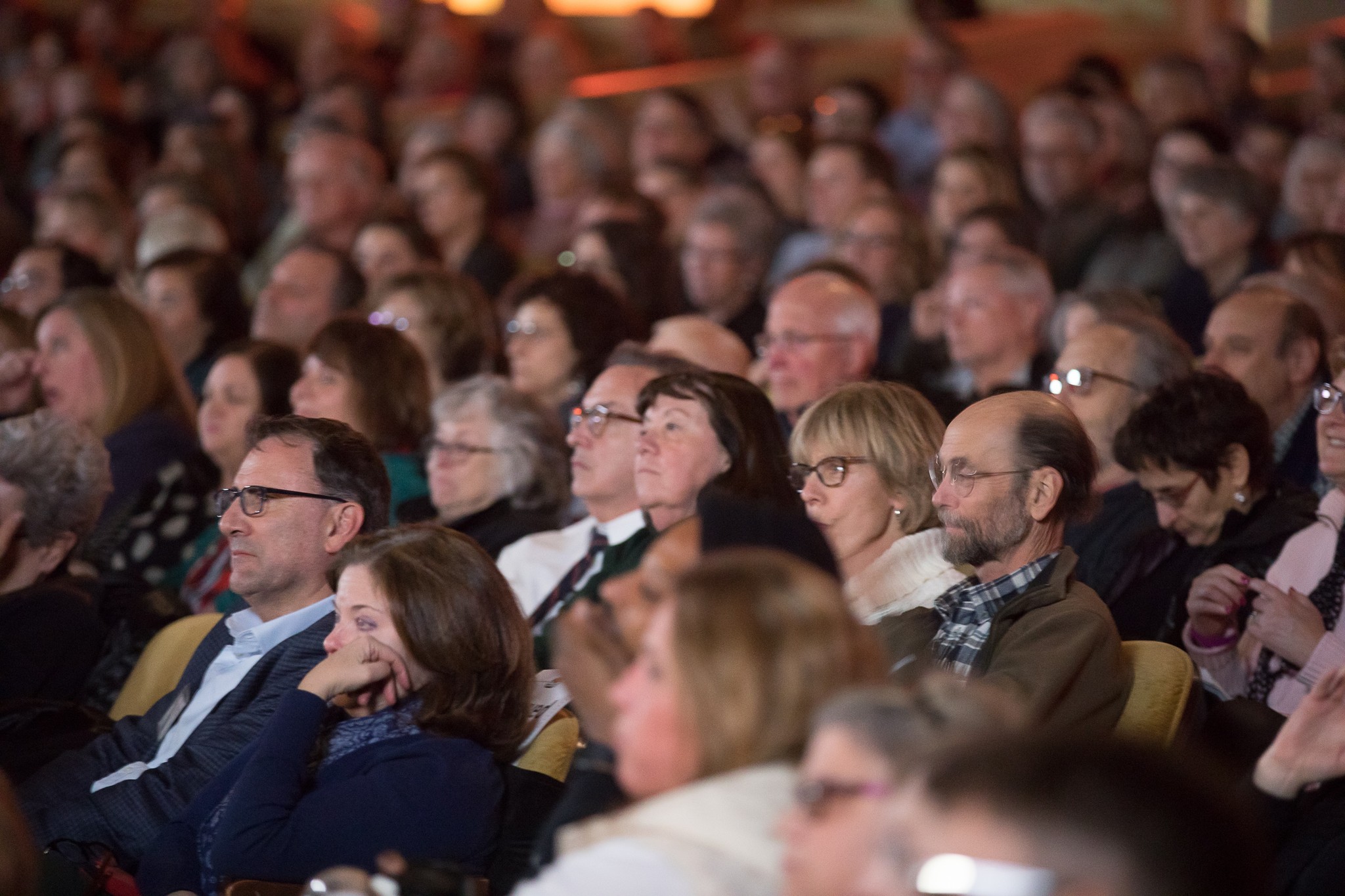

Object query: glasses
[1152,471,1202,508]
[567,405,638,438]
[1312,379,1345,415]
[213,484,346,519]
[927,453,1032,499]
[786,455,867,489]
[795,782,886,820]
[752,329,849,358]
[1040,365,1143,398]
[421,437,500,466]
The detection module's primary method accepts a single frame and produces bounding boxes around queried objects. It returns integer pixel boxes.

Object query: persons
[491,337,703,640]
[783,377,975,688]
[528,359,791,706]
[1083,360,1314,714]
[0,1,1345,896]
[868,386,1135,775]
[19,408,393,887]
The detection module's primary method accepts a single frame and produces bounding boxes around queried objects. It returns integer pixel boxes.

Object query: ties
[522,530,608,631]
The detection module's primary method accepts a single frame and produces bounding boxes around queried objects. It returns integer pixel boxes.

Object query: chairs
[106,611,224,722]
[1114,639,1194,745]
